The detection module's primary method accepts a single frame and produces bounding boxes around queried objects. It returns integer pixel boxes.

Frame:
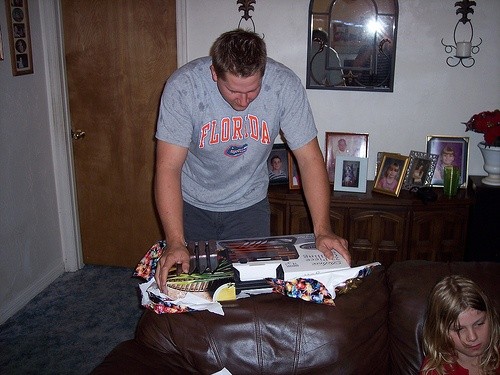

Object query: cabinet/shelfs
[271,187,479,268]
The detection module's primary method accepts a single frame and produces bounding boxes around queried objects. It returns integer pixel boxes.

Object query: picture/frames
[265,144,301,190]
[4,0,34,76]
[326,130,471,197]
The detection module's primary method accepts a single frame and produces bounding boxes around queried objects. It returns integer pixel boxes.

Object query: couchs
[94,260,500,375]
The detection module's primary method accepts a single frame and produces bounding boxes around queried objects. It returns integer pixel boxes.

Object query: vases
[480,142,500,187]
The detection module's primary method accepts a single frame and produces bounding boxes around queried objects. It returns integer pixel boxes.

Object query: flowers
[465,108,500,149]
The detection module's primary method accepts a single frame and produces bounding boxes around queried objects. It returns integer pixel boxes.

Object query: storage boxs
[215,233,350,282]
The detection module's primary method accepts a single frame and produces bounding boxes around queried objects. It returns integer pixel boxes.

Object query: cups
[444,166,460,196]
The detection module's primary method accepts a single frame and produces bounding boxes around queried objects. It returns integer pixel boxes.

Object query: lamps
[440,1,482,68]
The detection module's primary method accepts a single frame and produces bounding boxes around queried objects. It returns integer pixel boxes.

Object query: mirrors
[306,0,400,93]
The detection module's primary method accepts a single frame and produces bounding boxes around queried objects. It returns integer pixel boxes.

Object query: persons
[412,164,424,185]
[310,30,346,86]
[378,161,400,190]
[13,0,24,67]
[352,28,392,87]
[154,29,351,294]
[269,155,301,185]
[435,147,458,180]
[420,274,500,375]
[334,139,354,186]
